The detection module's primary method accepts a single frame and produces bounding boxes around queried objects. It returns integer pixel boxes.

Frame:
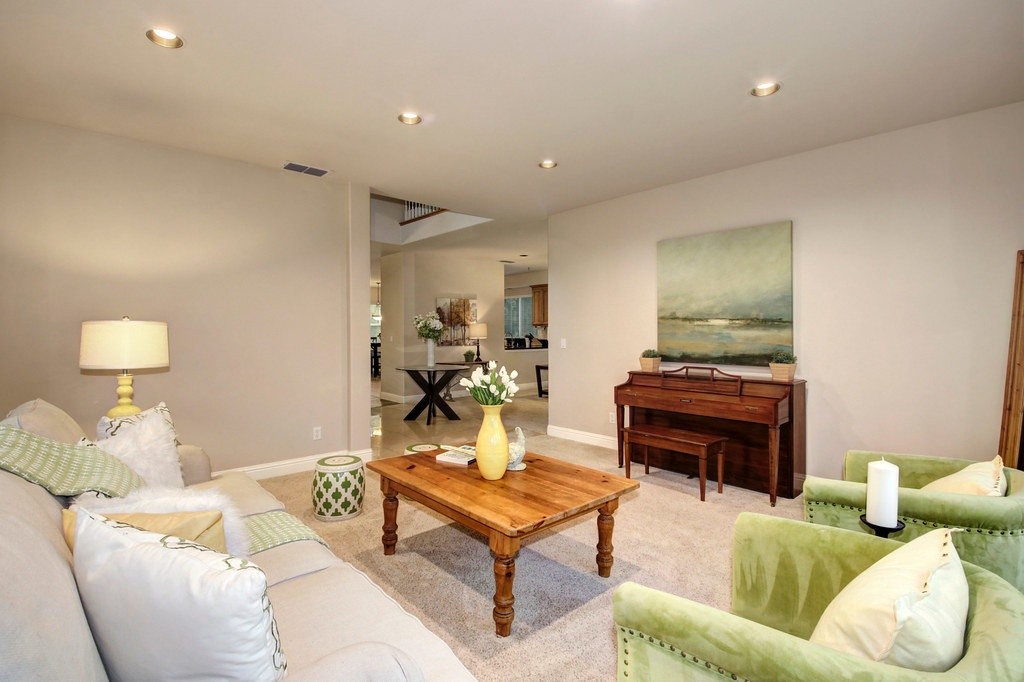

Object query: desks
[614,371,807,508]
[395,360,498,426]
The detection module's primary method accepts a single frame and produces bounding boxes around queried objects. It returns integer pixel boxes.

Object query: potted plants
[463,350,475,362]
[769,350,798,382]
[639,348,663,371]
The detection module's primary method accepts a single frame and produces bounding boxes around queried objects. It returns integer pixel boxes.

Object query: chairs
[370,342,381,375]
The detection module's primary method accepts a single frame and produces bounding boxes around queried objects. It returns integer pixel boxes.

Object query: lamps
[468,324,487,361]
[80,315,170,418]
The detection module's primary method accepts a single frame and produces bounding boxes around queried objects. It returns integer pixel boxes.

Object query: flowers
[412,310,446,340]
[460,359,520,406]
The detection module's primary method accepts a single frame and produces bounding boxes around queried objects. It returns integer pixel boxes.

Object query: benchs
[621,424,729,502]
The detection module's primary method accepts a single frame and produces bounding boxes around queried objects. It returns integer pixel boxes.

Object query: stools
[311,454,365,522]
[404,443,441,500]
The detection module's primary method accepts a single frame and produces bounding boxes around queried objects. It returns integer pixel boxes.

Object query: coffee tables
[366,441,640,636]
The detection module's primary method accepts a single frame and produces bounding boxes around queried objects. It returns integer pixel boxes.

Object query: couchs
[802,449,1024,598]
[0,400,475,682]
[613,511,1024,682]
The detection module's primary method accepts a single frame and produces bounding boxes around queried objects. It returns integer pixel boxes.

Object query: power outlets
[313,427,322,440]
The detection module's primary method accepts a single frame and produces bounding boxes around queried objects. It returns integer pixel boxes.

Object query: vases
[427,338,436,368]
[475,405,509,480]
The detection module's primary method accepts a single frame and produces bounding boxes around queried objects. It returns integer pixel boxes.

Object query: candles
[866,456,899,528]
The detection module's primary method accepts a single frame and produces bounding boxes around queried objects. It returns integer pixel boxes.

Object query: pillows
[921,455,1007,499]
[61,402,288,682]
[807,527,968,674]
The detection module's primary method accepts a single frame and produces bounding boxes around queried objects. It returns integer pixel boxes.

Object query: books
[436,445,476,466]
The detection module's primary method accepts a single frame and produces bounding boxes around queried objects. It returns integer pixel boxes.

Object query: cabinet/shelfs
[530,284,548,327]
